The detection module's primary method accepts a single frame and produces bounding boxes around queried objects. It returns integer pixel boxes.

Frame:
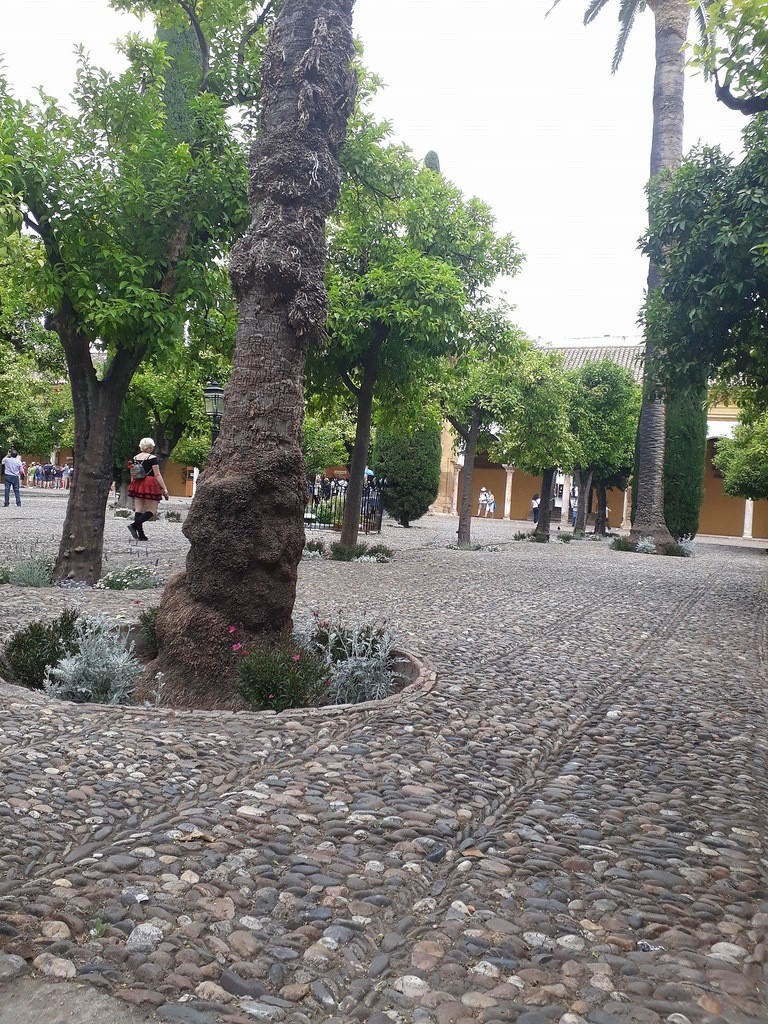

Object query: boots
[127,510,155,541]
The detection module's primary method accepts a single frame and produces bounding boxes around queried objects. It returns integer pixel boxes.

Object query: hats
[480,487,487,492]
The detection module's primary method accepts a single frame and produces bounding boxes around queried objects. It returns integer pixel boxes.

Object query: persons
[16,462,74,490]
[127,438,169,541]
[570,496,578,526]
[605,500,611,530]
[530,493,541,523]
[475,487,494,519]
[2,450,25,507]
[311,475,382,516]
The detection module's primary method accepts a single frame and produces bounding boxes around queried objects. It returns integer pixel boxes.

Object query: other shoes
[483,516,488,518]
[491,517,494,518]
[3,504,9,507]
[17,503,21,507]
[476,515,480,517]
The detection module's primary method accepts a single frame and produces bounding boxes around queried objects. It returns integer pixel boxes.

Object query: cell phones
[9,449,12,454]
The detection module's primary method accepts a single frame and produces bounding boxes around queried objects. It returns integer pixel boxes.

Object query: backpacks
[130,454,153,480]
[35,467,39,475]
